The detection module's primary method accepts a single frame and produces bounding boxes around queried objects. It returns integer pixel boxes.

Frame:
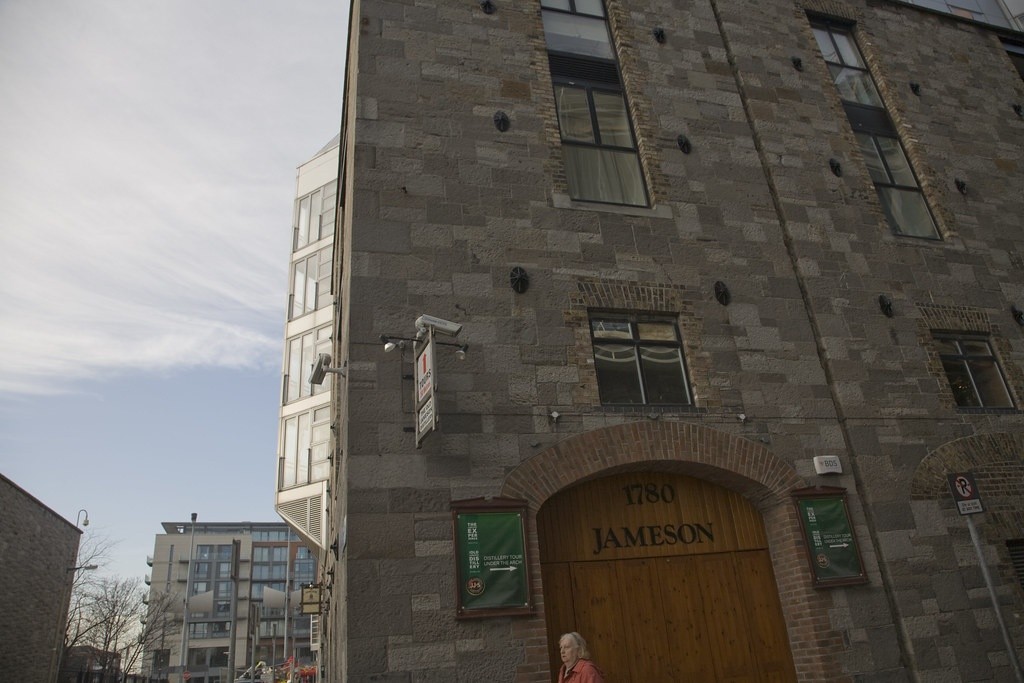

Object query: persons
[558,631,606,683]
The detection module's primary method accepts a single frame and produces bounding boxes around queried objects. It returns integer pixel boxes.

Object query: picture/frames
[447,495,538,621]
[790,484,873,591]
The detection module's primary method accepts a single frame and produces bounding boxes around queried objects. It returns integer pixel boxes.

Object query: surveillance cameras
[422,314,463,338]
[308,354,331,385]
[82,520,89,527]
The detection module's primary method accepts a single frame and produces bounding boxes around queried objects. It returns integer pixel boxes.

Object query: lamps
[76,509,89,527]
[379,334,423,352]
[67,564,98,570]
[436,341,470,360]
